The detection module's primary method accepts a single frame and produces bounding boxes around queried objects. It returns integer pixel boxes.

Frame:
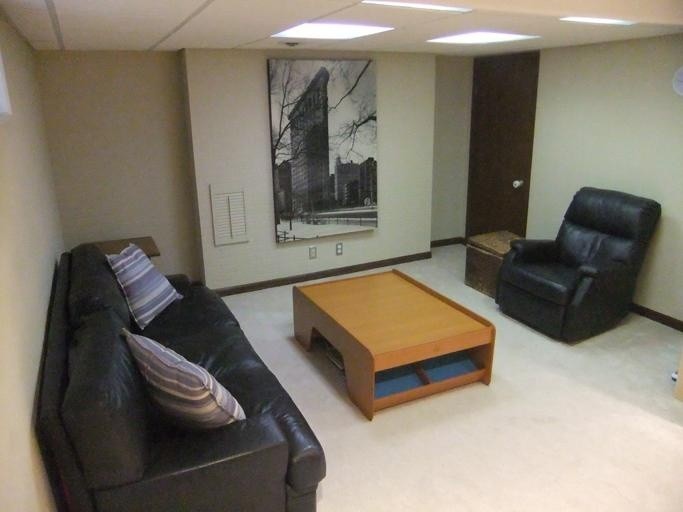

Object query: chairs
[499,185,660,345]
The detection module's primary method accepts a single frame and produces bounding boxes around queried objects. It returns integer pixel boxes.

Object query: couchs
[36,250,327,512]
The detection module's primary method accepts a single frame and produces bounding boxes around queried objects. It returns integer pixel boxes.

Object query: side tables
[73,235,160,261]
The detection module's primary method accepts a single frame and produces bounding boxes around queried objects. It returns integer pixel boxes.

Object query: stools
[465,231,526,299]
